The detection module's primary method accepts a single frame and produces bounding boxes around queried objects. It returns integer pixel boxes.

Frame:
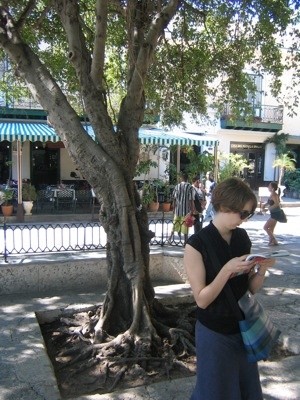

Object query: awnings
[0,121,219,223]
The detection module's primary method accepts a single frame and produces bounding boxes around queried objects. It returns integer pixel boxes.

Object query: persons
[165,174,197,245]
[184,177,277,400]
[191,170,217,235]
[261,181,287,246]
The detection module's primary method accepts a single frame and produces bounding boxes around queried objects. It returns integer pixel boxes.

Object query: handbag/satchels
[235,288,281,362]
[278,210,287,223]
[183,212,195,227]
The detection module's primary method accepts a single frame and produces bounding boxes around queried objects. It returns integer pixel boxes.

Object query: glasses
[239,209,255,220]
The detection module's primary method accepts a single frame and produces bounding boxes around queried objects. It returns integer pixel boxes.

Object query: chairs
[0,184,100,217]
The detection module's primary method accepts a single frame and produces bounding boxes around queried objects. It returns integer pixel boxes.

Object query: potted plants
[0,189,15,216]
[133,158,177,213]
[22,182,37,215]
[282,168,300,199]
